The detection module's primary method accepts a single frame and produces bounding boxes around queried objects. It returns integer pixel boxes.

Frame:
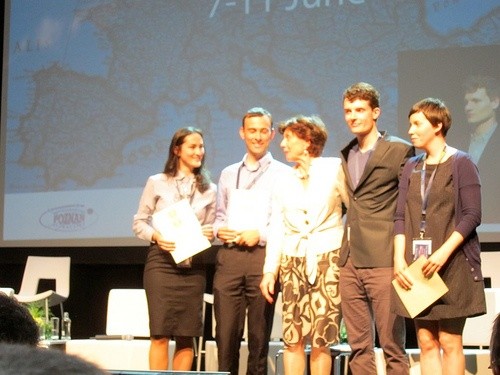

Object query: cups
[38,318,45,339]
[51,317,59,339]
[122,335,134,340]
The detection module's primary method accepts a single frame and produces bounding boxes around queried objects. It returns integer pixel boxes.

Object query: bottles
[61,312,71,340]
[340,318,347,345]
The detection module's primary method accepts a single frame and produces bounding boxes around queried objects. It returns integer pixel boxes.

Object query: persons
[392,97,482,375]
[339,82,417,375]
[457,80,500,224]
[214,109,291,375]
[132,127,219,371]
[260,114,355,375]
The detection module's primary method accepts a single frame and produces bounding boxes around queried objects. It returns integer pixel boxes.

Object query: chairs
[196,251,500,375]
[15,255,71,340]
